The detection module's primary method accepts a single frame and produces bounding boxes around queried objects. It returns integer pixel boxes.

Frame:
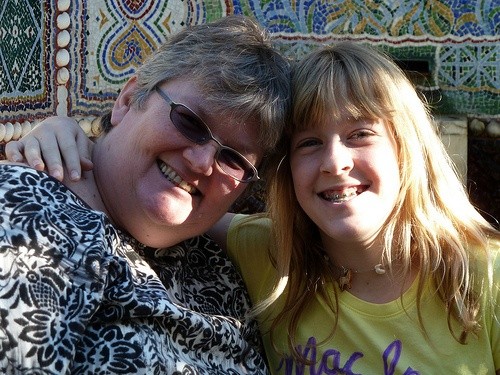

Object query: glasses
[150,81,265,186]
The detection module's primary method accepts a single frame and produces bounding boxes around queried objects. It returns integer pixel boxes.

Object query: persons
[3,41,500,374]
[1,15,298,375]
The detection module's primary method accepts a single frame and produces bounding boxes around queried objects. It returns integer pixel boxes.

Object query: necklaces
[320,245,407,292]
